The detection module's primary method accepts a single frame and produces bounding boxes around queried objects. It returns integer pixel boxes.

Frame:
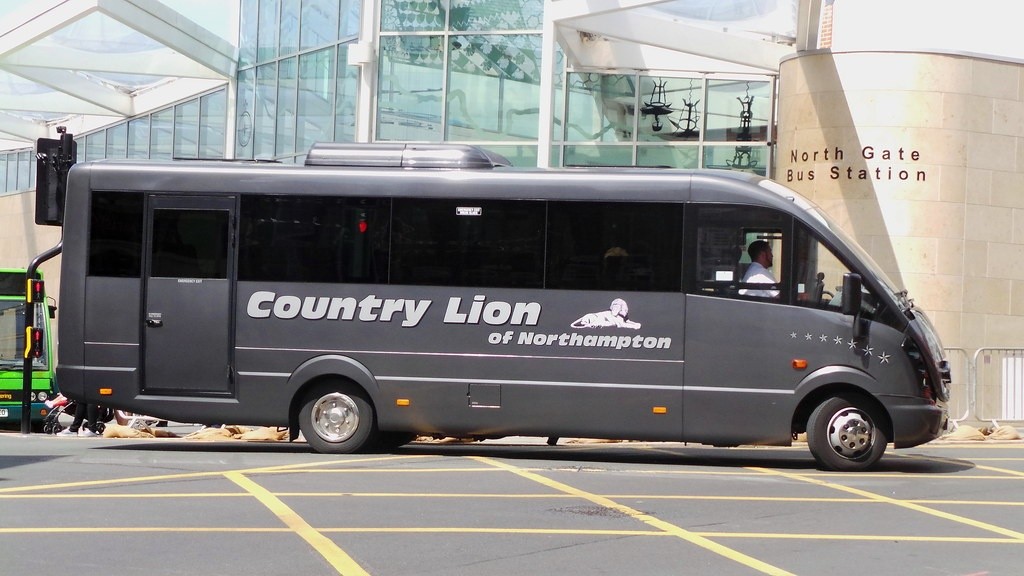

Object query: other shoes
[45,400,53,408]
[56,426,96,437]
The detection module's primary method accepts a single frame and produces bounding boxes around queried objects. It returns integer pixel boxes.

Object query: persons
[55,402,97,436]
[737,241,780,297]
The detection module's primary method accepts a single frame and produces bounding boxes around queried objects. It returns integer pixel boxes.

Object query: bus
[53,137,953,474]
[0,266,57,426]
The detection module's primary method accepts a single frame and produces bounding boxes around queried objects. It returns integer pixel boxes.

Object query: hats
[748,241,769,263]
